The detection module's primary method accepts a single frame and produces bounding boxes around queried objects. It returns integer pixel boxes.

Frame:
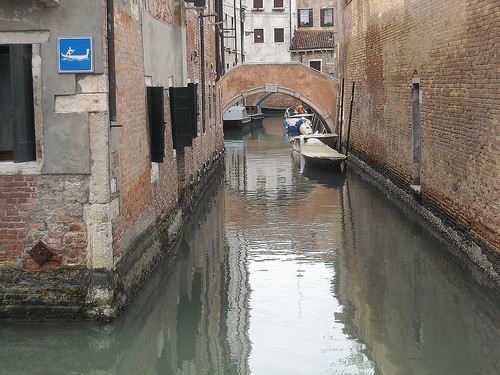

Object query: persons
[292,104,304,114]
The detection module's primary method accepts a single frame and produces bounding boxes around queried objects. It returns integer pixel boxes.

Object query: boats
[301,137,347,172]
[283,104,314,129]
[223,106,253,128]
[291,131,338,152]
[243,105,265,124]
